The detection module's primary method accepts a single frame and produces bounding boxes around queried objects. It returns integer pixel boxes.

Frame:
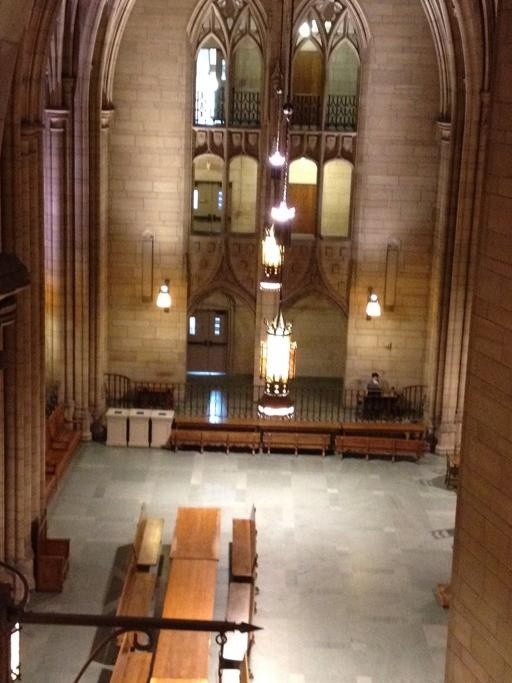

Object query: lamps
[155,278,174,313]
[269,102,297,225]
[267,0,287,167]
[258,276,283,291]
[255,309,299,419]
[365,284,383,322]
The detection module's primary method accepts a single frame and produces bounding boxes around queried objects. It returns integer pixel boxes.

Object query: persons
[364,372,385,420]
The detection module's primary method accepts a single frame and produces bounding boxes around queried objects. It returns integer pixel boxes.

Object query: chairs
[445,446,459,488]
[454,443,461,477]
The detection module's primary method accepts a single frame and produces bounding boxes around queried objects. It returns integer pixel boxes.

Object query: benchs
[215,653,252,682]
[132,501,167,579]
[108,632,154,682]
[110,546,157,648]
[171,413,429,464]
[230,502,259,583]
[217,578,259,666]
[45,399,84,505]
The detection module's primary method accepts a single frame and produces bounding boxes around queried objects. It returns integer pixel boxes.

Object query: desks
[147,629,212,682]
[134,385,176,409]
[356,389,398,418]
[450,454,461,465]
[165,504,222,562]
[159,557,219,625]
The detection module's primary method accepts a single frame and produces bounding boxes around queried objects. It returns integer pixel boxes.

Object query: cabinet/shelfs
[30,507,72,597]
